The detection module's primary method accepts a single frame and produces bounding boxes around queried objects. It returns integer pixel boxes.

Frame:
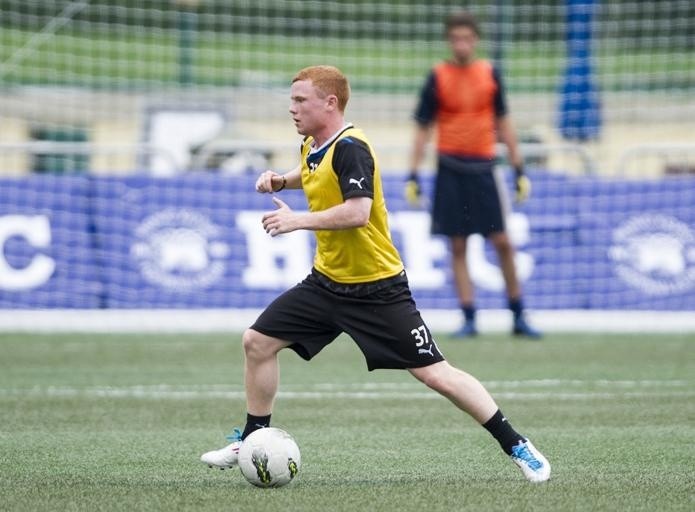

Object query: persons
[196,70,553,489]
[403,14,544,338]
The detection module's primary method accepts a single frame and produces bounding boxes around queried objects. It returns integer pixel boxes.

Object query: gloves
[514,169,530,203]
[405,173,421,208]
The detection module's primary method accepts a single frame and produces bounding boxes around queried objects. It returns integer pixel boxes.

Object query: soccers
[239,427,299,488]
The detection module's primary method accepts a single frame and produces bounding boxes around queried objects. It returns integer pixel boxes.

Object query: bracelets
[274,175,287,193]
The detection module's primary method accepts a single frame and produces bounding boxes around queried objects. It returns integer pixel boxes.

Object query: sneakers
[200,438,243,471]
[512,437,551,482]
[449,319,478,338]
[513,317,542,339]
